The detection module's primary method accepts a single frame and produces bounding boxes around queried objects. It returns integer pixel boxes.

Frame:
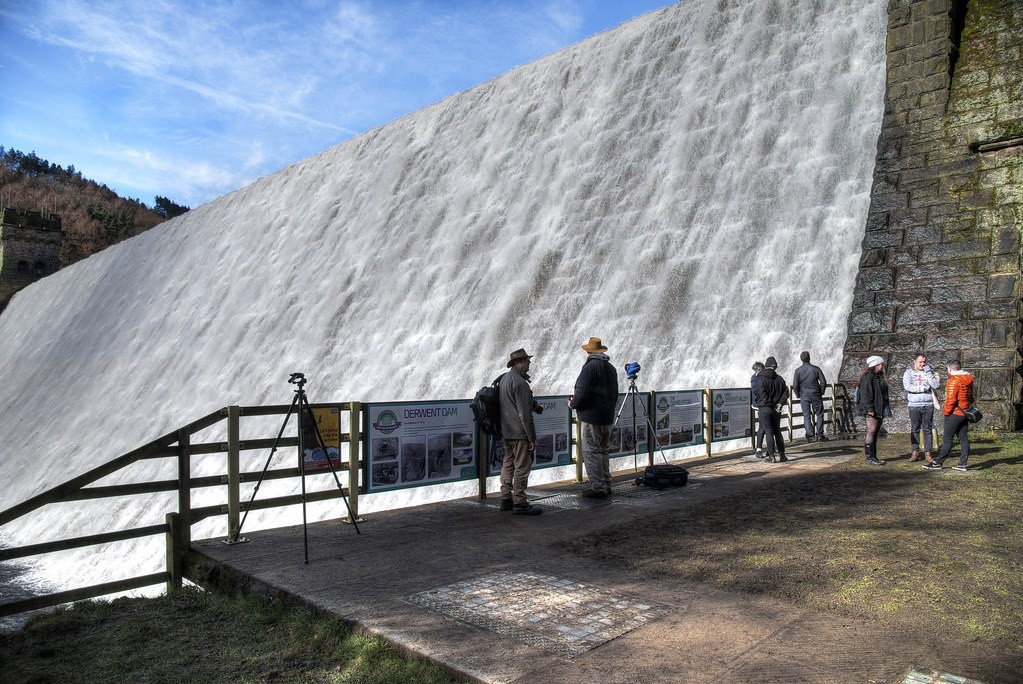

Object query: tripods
[608,375,669,471]
[233,385,362,564]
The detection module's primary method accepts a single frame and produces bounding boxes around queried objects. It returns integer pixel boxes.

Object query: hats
[582,337,608,352]
[507,348,534,368]
[765,357,777,368]
[867,356,884,367]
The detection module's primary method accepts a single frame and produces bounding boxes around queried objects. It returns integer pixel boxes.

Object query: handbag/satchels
[919,422,939,452]
[930,388,940,410]
[636,464,688,490]
[964,403,983,423]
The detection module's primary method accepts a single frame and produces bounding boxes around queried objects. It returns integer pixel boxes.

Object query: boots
[864,444,885,464]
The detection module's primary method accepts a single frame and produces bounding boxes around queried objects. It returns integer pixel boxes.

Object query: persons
[903,353,940,463]
[857,355,892,464]
[751,357,790,463]
[793,351,830,441]
[568,337,619,497]
[498,348,543,515]
[922,359,975,471]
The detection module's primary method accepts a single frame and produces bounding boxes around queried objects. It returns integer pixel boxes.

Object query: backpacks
[470,372,506,436]
[495,386,497,387]
[854,384,860,402]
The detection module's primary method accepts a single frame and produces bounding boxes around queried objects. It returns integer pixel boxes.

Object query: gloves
[925,385,931,393]
[923,365,930,372]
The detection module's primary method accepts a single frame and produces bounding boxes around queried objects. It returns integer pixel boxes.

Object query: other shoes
[908,455,919,461]
[581,489,607,498]
[769,457,776,462]
[500,503,513,510]
[807,437,813,442]
[766,451,774,456]
[780,455,788,462]
[513,504,543,514]
[756,451,764,458]
[607,488,612,494]
[816,434,828,441]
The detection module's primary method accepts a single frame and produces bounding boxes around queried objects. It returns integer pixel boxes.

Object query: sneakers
[952,465,967,471]
[921,461,942,470]
